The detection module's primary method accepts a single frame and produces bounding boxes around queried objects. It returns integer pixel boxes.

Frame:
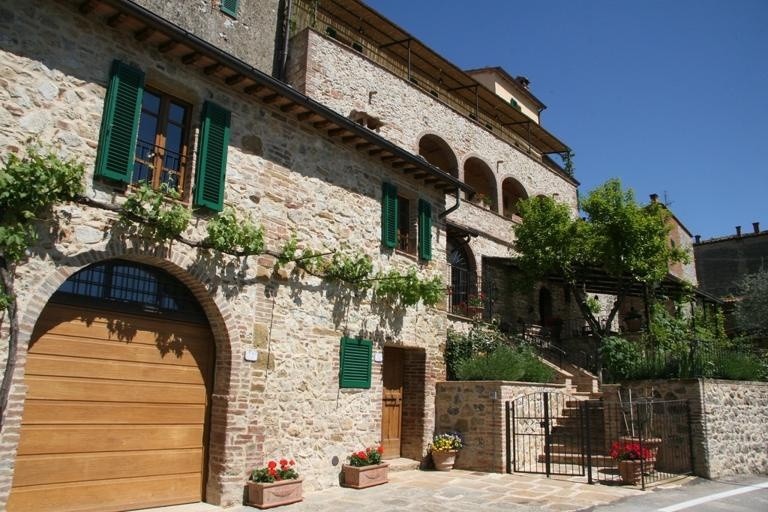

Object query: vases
[618,436,662,475]
[484,201,490,210]
[476,198,483,207]
[467,305,485,314]
[246,480,303,509]
[430,450,458,471]
[341,464,390,489]
[621,459,635,485]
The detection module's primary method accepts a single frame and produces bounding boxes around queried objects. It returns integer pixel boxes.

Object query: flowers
[474,191,484,201]
[348,447,386,466]
[427,431,465,456]
[464,291,485,307]
[609,440,652,461]
[484,195,492,204]
[250,459,300,483]
